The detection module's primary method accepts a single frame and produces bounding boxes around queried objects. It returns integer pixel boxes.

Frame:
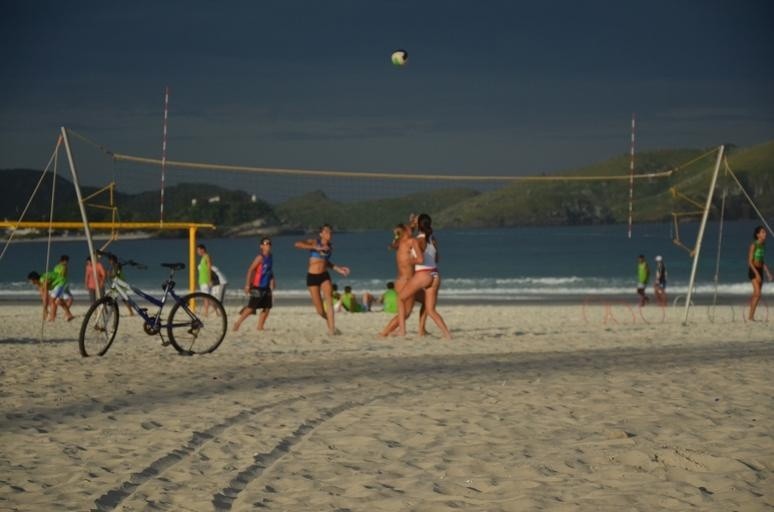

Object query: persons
[197,263,228,317]
[28,270,73,322]
[636,253,650,307]
[293,213,453,340]
[653,255,667,306]
[41,255,73,312]
[748,226,773,319]
[232,237,275,332]
[84,256,108,318]
[196,244,213,318]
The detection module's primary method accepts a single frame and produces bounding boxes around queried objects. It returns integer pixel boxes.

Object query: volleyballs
[392,50,408,66]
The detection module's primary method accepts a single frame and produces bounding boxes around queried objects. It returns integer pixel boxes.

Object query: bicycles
[77,248,228,358]
